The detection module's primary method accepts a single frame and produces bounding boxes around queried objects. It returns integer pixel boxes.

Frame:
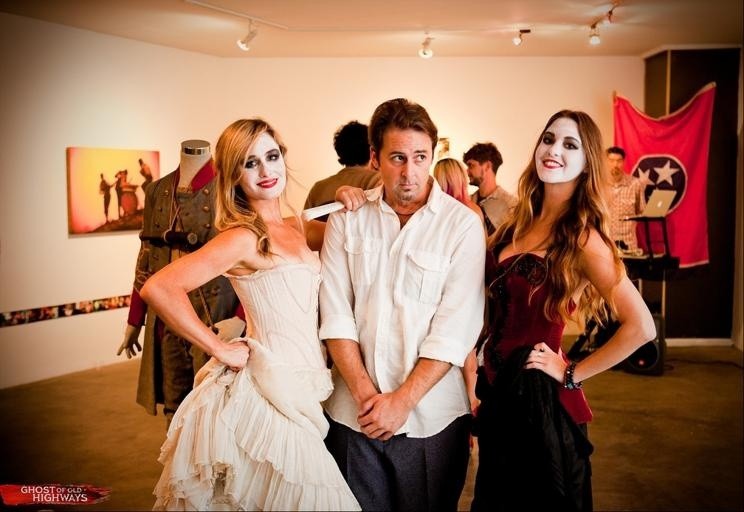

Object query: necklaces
[166,169,180,231]
[395,210,414,217]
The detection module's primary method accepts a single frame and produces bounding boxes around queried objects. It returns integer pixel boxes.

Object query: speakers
[622,313,665,377]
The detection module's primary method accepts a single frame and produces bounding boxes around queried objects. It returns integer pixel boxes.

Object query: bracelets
[563,361,584,397]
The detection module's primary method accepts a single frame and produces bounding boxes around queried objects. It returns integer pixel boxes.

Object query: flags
[612,79,716,267]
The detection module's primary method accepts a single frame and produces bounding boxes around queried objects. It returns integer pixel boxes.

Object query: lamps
[513,29,531,47]
[418,35,436,59]
[237,14,262,53]
[587,1,622,45]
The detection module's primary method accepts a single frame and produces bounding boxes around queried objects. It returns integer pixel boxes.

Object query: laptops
[619,190,677,220]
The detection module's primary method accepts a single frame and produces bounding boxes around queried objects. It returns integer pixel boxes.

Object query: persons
[138,157,154,194]
[601,147,649,254]
[478,111,655,510]
[135,115,365,510]
[96,172,115,226]
[461,142,519,254]
[320,97,489,508]
[433,157,489,450]
[115,167,129,219]
[115,140,248,430]
[302,123,384,227]
